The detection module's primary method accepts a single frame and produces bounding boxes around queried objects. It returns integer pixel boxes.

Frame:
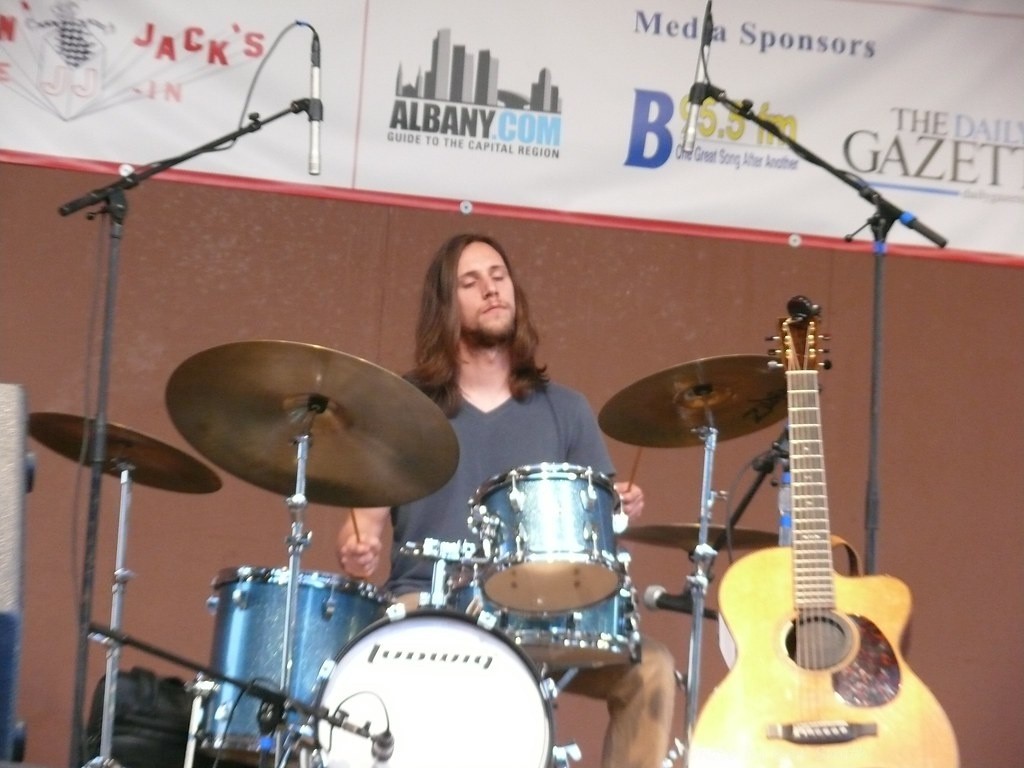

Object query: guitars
[685,316,961,768]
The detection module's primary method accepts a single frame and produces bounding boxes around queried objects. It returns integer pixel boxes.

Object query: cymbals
[164,340,461,509]
[617,521,781,553]
[25,410,224,495]
[597,352,788,449]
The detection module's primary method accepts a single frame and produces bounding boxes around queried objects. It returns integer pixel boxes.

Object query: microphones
[682,19,713,153]
[309,34,321,176]
[371,726,394,762]
[642,585,718,622]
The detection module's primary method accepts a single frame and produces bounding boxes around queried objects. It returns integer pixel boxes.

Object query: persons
[338,234,675,768]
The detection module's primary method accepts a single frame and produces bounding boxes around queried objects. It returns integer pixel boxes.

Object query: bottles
[778,473,794,546]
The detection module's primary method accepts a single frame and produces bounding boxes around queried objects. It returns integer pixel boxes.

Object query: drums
[447,574,643,670]
[469,460,627,615]
[296,608,558,768]
[194,563,398,766]
